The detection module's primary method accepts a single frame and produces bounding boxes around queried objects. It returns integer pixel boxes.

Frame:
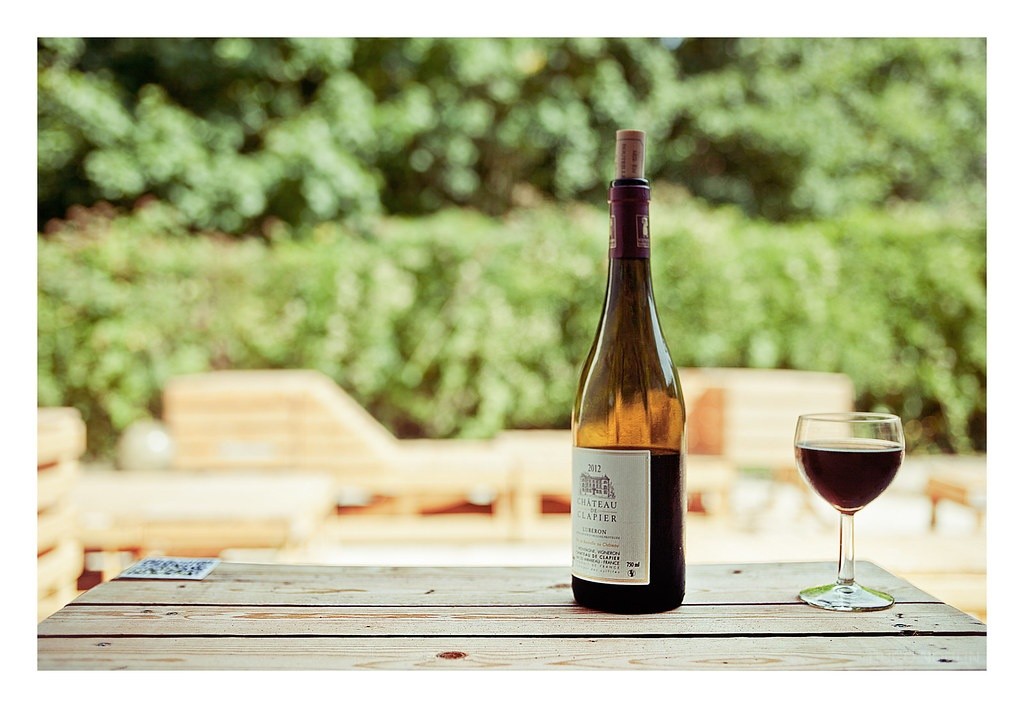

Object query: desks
[38,560,986,669]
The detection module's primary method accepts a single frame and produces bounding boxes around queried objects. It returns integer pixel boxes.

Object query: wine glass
[794,412,905,612]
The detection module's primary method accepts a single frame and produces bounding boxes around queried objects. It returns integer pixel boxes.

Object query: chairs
[35,366,988,622]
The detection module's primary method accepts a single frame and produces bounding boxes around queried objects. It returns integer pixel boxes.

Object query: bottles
[572,131,685,614]
